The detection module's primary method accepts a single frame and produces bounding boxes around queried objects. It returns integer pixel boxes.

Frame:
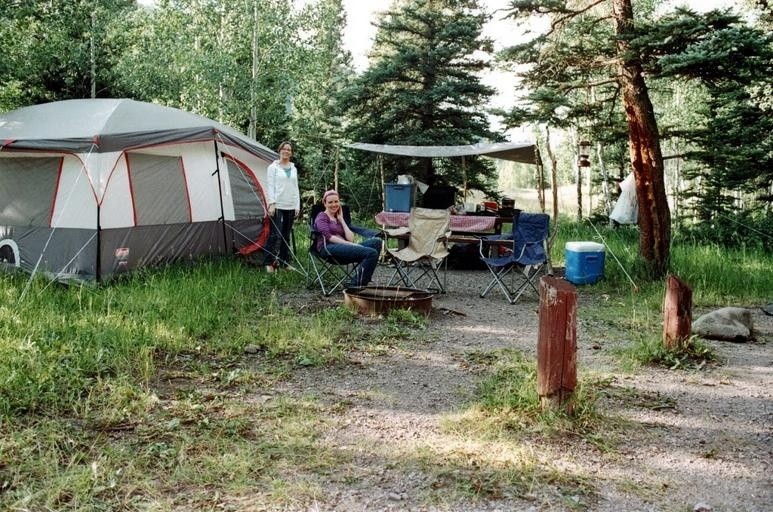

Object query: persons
[312,189,383,288]
[262,140,301,275]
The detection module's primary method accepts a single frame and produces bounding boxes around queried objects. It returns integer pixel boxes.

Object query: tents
[0,96,283,292]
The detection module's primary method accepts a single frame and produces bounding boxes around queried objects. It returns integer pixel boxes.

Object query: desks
[374,211,515,266]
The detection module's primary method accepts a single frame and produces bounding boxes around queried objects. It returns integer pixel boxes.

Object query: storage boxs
[565,240,606,285]
[383,181,415,212]
[421,186,457,209]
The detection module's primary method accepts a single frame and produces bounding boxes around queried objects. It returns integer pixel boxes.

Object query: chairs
[478,211,551,306]
[306,202,362,296]
[378,204,452,296]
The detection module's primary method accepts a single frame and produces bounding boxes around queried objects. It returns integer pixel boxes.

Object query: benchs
[377,229,513,263]
